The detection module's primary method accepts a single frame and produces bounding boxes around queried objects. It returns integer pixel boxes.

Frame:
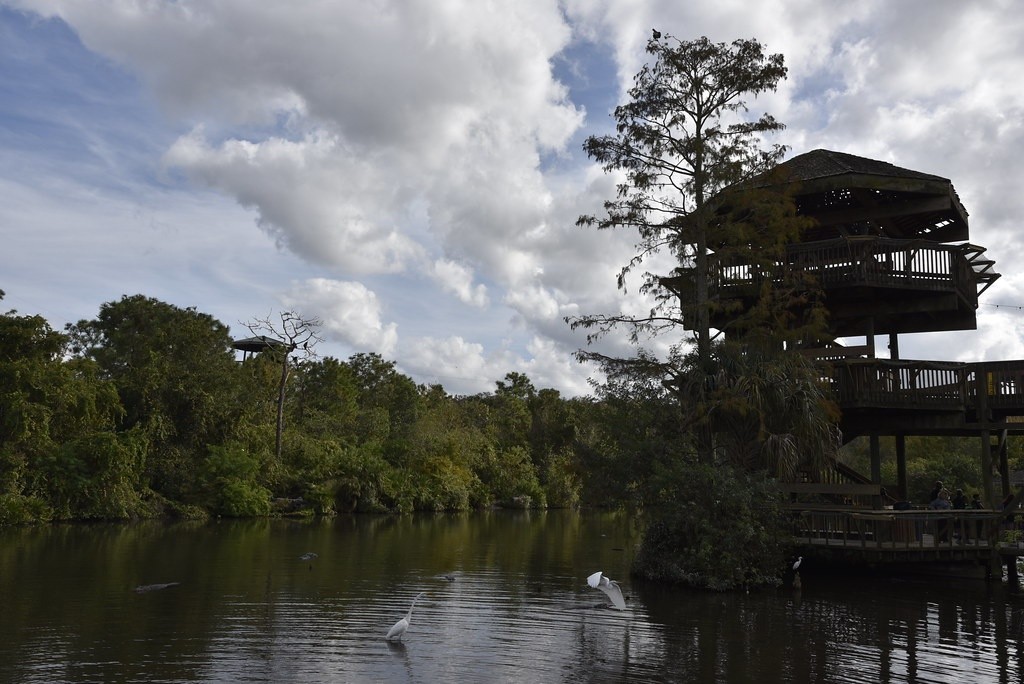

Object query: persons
[1001,495,1024,540]
[972,494,984,540]
[929,481,973,544]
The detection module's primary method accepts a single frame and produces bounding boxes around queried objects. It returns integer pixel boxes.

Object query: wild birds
[297,552,319,560]
[385,591,424,642]
[587,571,627,612]
[793,556,803,570]
